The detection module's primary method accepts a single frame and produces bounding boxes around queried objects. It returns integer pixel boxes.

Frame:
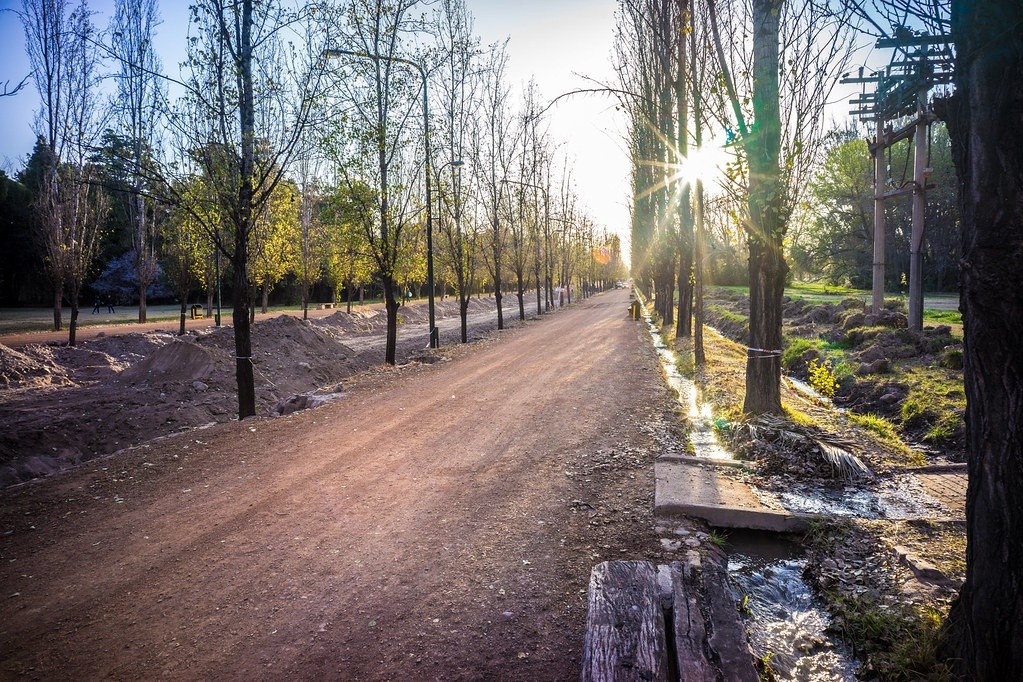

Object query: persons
[106,295,115,313]
[407,288,410,302]
[584,278,588,298]
[92,295,100,314]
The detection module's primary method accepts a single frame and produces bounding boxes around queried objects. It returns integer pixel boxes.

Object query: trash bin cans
[632,300,641,321]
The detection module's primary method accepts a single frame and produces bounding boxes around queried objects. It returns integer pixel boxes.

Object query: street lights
[317,42,439,346]
[424,157,465,347]
[498,175,550,313]
